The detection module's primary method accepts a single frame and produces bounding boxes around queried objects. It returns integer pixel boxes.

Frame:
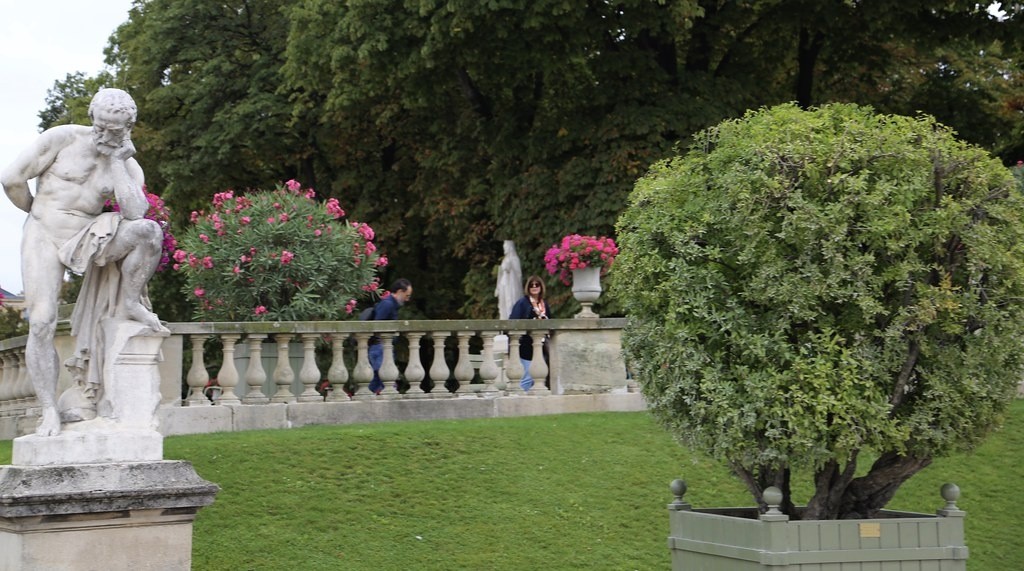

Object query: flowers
[206,378,220,388]
[544,234,619,286]
[104,183,178,273]
[172,180,390,344]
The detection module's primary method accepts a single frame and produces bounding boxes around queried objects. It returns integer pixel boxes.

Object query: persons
[0,89,164,437]
[367,278,413,395]
[502,275,554,390]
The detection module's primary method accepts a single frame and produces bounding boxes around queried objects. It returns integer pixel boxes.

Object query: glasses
[529,283,540,288]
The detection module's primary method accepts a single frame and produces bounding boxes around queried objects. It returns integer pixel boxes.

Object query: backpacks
[358,299,390,321]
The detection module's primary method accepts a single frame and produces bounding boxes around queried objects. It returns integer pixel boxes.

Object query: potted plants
[606,100,1024,570]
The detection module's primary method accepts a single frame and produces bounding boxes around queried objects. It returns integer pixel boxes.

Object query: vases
[570,269,603,303]
[232,344,307,400]
[212,388,221,399]
